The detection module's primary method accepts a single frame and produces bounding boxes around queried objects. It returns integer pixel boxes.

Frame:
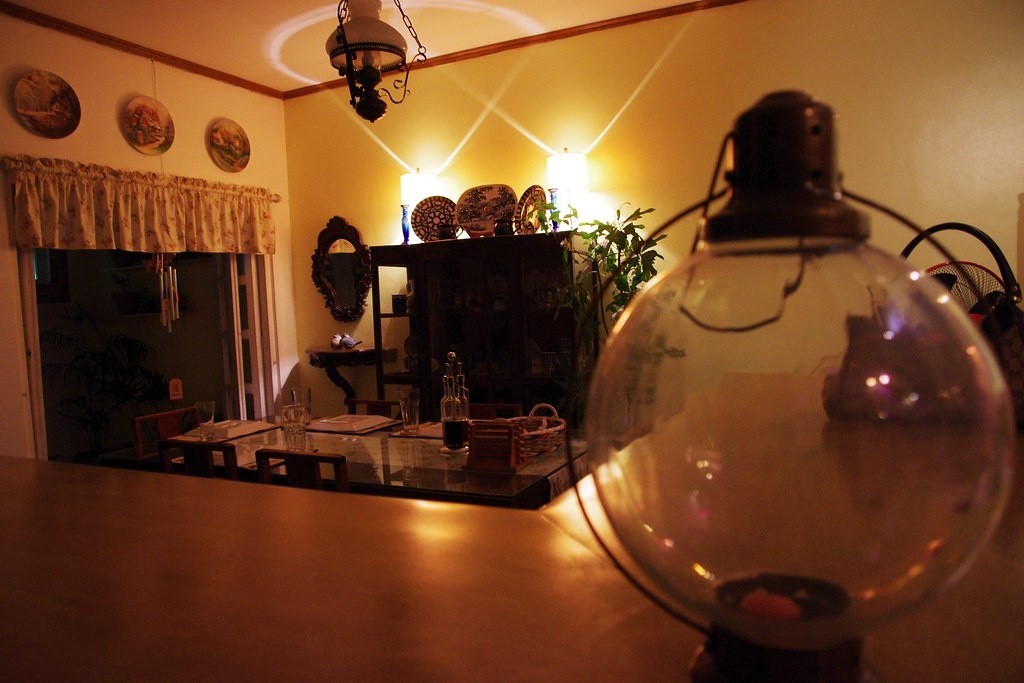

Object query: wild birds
[330,332,363,350]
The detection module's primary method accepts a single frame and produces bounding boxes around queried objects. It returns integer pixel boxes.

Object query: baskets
[495,403,567,464]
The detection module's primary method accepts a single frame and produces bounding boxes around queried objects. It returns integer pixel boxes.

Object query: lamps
[325,0,426,122]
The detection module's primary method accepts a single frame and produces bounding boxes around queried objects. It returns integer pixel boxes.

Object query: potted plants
[40,303,165,466]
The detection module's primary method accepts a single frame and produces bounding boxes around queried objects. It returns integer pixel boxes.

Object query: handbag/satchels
[900,222,1023,426]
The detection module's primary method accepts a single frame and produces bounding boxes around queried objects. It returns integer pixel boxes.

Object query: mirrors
[311,216,371,322]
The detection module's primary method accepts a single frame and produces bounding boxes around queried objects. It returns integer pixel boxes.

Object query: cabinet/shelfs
[369,232,593,424]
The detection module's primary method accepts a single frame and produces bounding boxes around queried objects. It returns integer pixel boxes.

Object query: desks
[306,347,397,414]
[172,416,591,496]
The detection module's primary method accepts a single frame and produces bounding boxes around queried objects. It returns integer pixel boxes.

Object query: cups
[398,388,419,432]
[392,295,406,314]
[195,400,215,442]
[281,404,306,452]
[291,386,311,424]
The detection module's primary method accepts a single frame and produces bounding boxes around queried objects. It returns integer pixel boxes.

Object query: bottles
[441,376,448,446]
[457,374,468,442]
[444,376,463,450]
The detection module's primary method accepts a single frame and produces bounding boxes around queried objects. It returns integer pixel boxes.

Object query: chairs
[133,403,522,498]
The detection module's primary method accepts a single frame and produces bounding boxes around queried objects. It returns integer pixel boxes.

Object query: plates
[209,119,250,172]
[455,184,518,232]
[306,415,393,431]
[14,71,81,139]
[185,419,272,437]
[411,197,460,243]
[123,97,174,156]
[515,185,547,234]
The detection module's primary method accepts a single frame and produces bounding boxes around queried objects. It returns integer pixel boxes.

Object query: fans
[926,261,1005,314]
